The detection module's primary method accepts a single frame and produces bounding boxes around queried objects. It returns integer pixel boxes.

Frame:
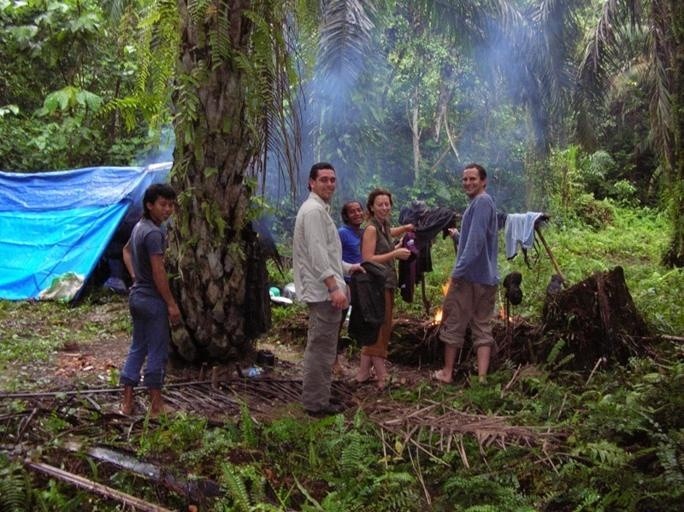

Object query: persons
[293,163,367,415]
[356,188,415,392]
[330,200,414,375]
[119,182,182,417]
[432,163,499,385]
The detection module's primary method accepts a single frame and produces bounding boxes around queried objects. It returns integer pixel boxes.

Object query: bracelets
[328,285,339,293]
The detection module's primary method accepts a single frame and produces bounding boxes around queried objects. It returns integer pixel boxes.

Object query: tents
[0,162,174,304]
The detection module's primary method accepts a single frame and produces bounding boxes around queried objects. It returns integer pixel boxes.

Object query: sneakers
[308,395,344,417]
[503,271,522,305]
[547,274,562,295]
